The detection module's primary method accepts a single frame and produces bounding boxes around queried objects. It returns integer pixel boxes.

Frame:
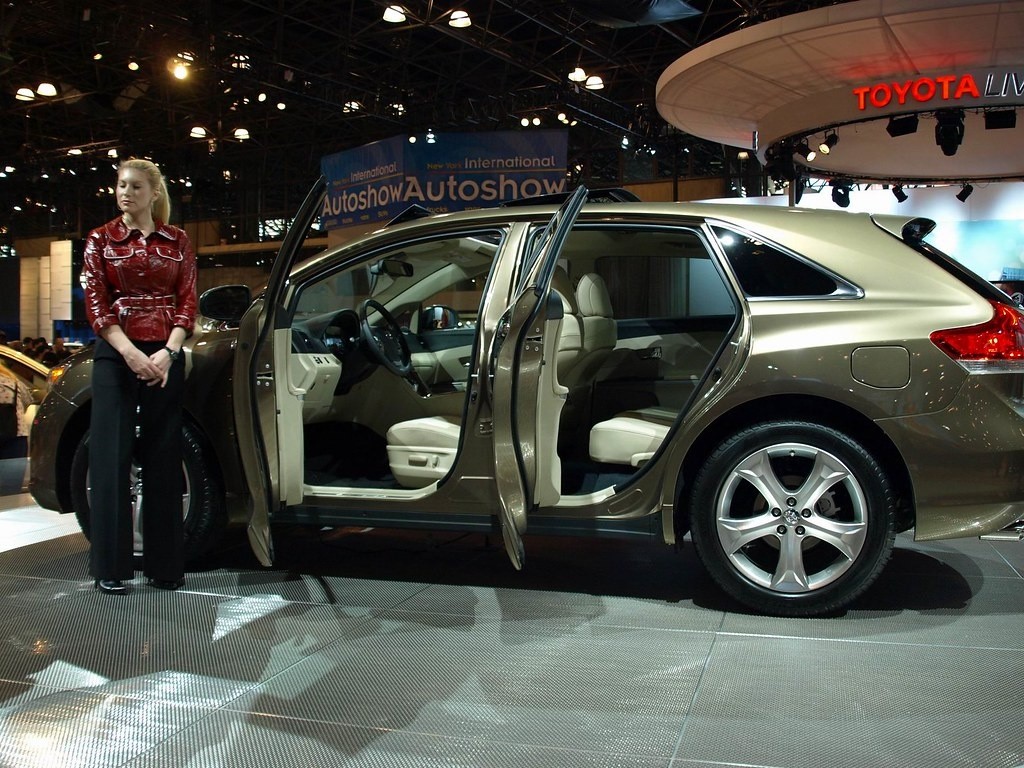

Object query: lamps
[0,4,755,260]
[764,100,1018,208]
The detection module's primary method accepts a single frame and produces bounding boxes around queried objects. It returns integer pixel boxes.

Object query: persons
[87,159,198,596]
[0,331,73,368]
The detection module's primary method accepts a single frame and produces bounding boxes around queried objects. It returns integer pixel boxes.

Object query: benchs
[589,389,694,461]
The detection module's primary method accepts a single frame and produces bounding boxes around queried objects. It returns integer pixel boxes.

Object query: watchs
[163,346,178,361]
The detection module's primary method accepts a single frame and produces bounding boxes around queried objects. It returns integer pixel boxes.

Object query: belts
[110,295,177,322]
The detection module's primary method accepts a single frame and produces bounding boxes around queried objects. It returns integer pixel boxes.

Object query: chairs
[578,275,619,360]
[388,274,584,486]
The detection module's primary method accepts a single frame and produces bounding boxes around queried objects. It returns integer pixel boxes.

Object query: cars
[0,345,50,437]
[27,175,1024,618]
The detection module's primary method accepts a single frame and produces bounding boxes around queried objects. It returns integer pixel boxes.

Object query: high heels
[148,575,178,590]
[93,576,126,594]
